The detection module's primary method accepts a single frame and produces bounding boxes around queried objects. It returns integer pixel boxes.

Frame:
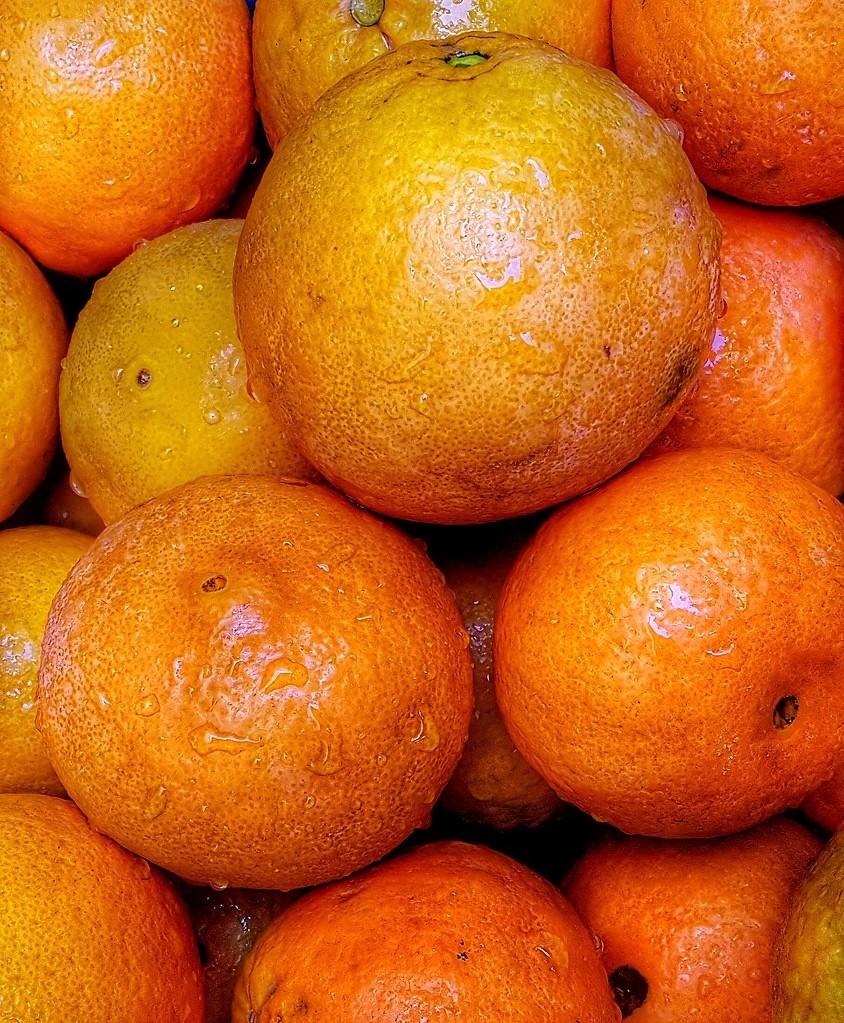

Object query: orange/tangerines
[0,0,844,1023]
